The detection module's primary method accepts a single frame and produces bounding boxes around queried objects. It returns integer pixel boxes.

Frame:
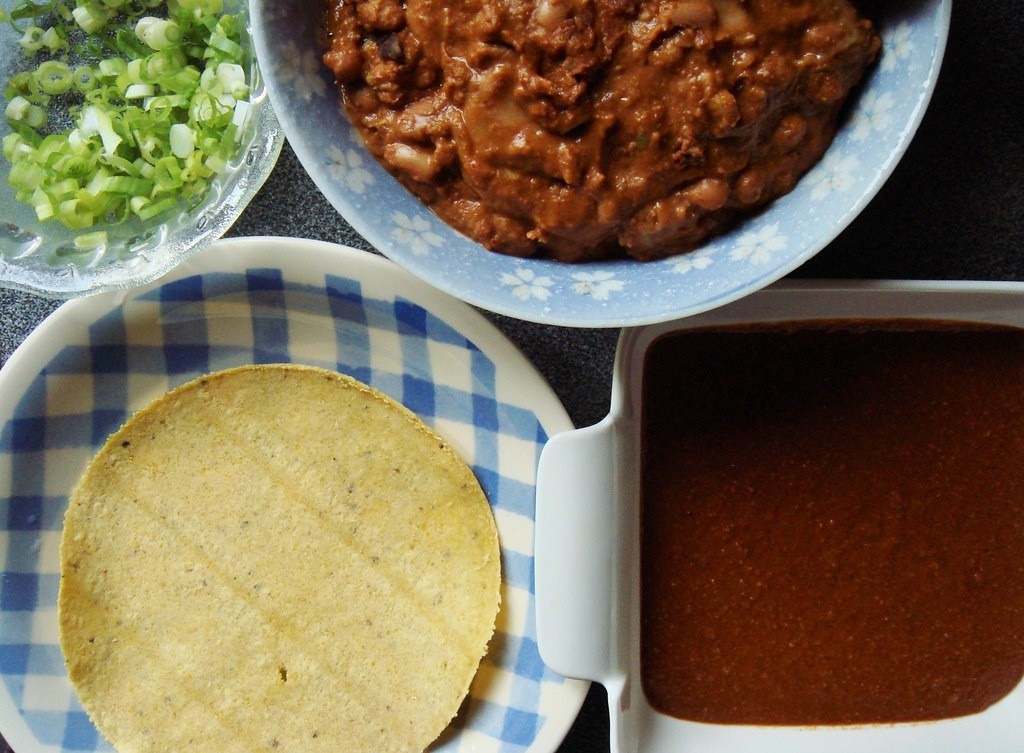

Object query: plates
[0,234,592,751]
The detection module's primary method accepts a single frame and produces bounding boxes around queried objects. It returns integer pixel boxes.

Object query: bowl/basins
[1,0,288,300]
[533,280,1024,751]
[249,0,952,328]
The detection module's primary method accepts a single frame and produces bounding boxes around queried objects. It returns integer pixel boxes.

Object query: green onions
[2,0,250,228]
